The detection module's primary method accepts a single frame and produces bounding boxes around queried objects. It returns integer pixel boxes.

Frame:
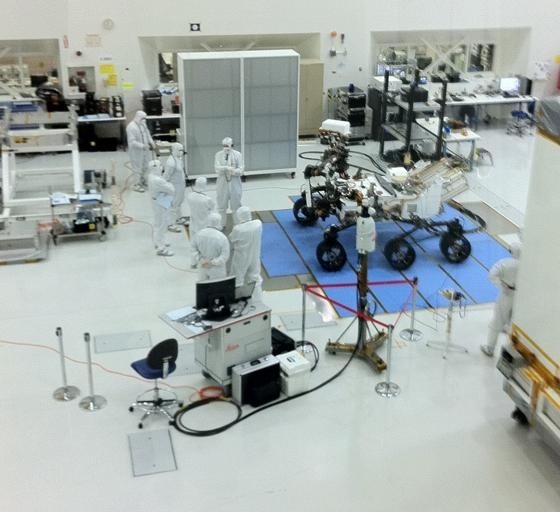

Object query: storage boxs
[275,350,311,397]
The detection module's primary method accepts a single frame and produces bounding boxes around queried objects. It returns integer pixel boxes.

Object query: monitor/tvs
[195,275,238,322]
[501,77,519,97]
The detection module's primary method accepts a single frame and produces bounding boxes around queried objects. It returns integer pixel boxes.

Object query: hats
[237,207,251,222]
[510,241,522,258]
[148,161,163,175]
[172,143,183,157]
[207,214,223,231]
[222,137,233,146]
[135,110,146,123]
[195,177,207,193]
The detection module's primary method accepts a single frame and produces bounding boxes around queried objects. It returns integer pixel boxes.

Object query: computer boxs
[514,74,532,95]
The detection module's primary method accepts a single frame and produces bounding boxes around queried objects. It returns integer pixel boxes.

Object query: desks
[415,92,538,172]
[77,114,127,151]
[145,112,181,142]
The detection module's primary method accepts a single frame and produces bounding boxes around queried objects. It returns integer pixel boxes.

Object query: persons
[479,242,521,357]
[164,143,189,233]
[147,160,176,255]
[228,205,263,303]
[189,212,232,282]
[126,110,157,193]
[214,136,245,232]
[186,176,215,240]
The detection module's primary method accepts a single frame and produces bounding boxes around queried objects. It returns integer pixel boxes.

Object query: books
[495,322,560,431]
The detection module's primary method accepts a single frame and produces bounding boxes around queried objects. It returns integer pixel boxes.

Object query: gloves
[143,144,150,151]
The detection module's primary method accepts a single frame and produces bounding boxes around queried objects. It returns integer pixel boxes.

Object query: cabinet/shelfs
[379,69,448,171]
[298,61,324,137]
[158,300,272,397]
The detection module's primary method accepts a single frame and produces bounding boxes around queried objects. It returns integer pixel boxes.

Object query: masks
[141,120,146,124]
[224,148,229,154]
[180,151,184,157]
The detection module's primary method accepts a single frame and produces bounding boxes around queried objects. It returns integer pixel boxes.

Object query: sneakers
[168,225,181,233]
[156,244,173,256]
[176,217,190,225]
[141,182,148,189]
[481,345,494,356]
[133,185,145,192]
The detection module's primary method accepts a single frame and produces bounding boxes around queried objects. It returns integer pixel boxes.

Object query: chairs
[129,339,183,428]
